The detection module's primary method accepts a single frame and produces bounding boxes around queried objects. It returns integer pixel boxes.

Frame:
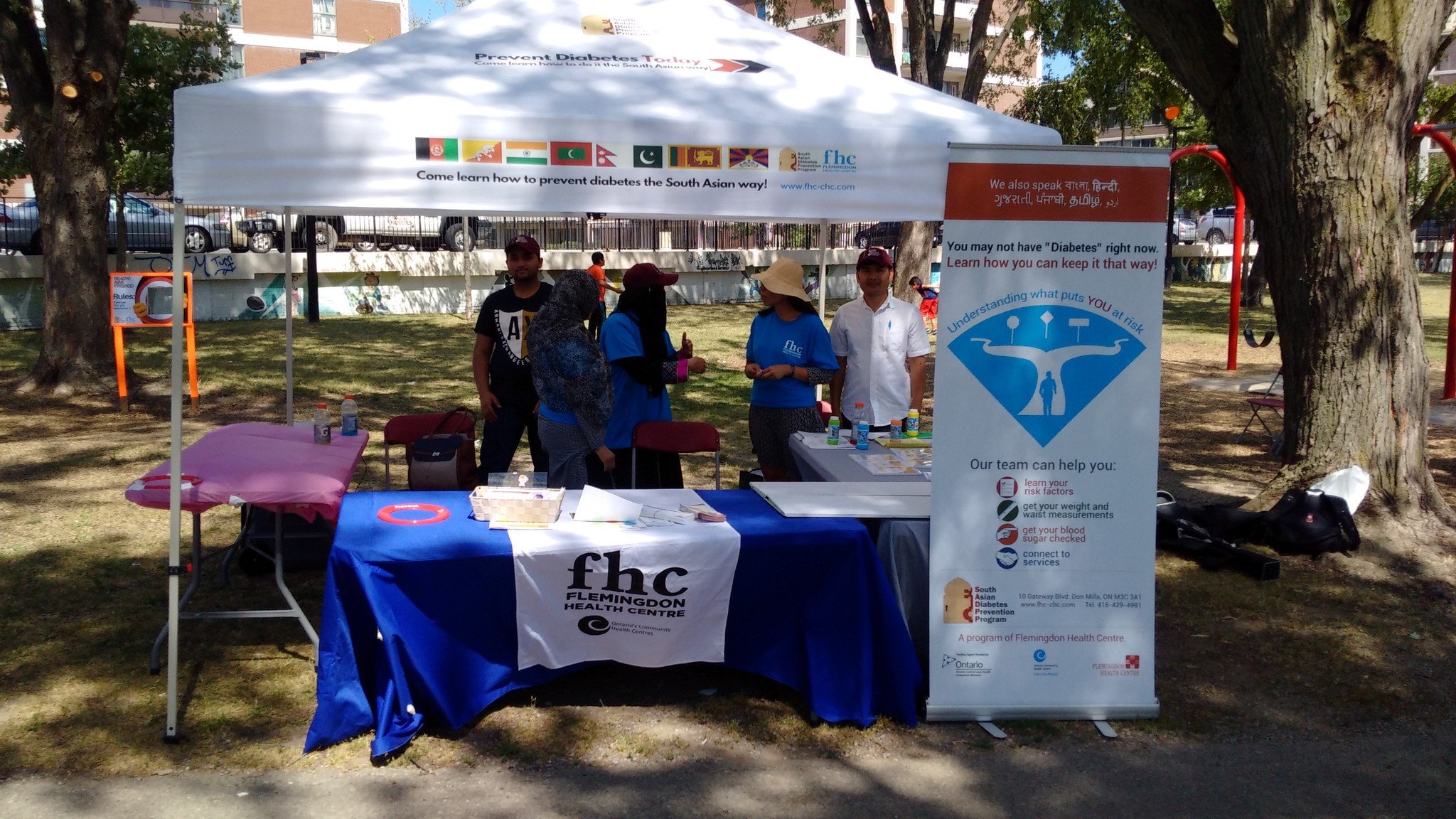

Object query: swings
[1242,196,1276,348]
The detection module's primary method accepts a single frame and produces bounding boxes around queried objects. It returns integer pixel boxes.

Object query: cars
[856,222,944,248]
[1,193,230,254]
[206,207,478,251]
[1168,218,1197,245]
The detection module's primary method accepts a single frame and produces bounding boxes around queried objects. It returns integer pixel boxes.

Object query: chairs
[632,421,720,490]
[384,413,475,490]
[1234,366,1284,444]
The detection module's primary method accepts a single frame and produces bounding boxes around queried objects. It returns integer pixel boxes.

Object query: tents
[165,0,1062,742]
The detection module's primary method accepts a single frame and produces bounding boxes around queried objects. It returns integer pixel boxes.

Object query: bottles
[850,402,866,444]
[856,421,869,450]
[827,416,840,444]
[907,409,919,436]
[890,417,902,442]
[341,395,357,436]
[313,404,331,445]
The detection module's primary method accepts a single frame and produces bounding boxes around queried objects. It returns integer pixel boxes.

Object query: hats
[857,247,894,271]
[623,263,678,291]
[504,236,540,256]
[750,258,811,301]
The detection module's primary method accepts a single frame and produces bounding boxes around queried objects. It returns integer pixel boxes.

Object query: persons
[744,257,839,482]
[828,248,932,433]
[473,233,706,488]
[909,276,940,335]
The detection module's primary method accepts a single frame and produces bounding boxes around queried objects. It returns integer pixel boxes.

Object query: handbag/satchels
[1262,487,1361,556]
[408,405,476,490]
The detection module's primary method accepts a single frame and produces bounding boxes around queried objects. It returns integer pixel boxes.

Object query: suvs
[1198,205,1254,244]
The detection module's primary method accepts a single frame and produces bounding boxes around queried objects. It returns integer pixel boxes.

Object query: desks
[303,490,919,758]
[125,423,370,672]
[789,431,929,667]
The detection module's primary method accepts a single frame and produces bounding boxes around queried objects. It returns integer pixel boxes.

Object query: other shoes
[926,327,930,332]
[929,329,937,335]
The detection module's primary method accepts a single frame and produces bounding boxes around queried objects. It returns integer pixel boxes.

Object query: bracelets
[791,365,795,377]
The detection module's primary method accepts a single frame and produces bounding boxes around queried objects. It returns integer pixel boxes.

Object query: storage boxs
[468,486,565,522]
